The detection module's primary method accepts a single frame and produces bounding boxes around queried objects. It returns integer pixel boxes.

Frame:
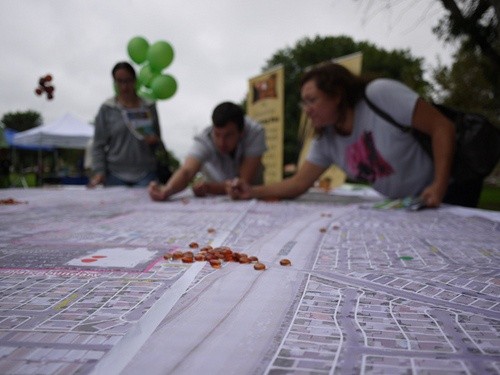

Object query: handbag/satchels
[361,78,500,186]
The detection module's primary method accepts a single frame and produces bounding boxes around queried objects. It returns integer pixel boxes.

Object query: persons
[148,100,268,202]
[86,61,166,189]
[224,62,485,210]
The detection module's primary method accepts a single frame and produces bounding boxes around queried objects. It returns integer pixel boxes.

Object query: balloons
[152,74,178,100]
[127,36,150,65]
[36,75,55,100]
[139,65,162,89]
[136,75,141,92]
[134,85,156,105]
[147,41,174,71]
[114,82,120,97]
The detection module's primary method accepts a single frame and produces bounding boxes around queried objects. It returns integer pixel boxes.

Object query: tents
[9,115,95,187]
